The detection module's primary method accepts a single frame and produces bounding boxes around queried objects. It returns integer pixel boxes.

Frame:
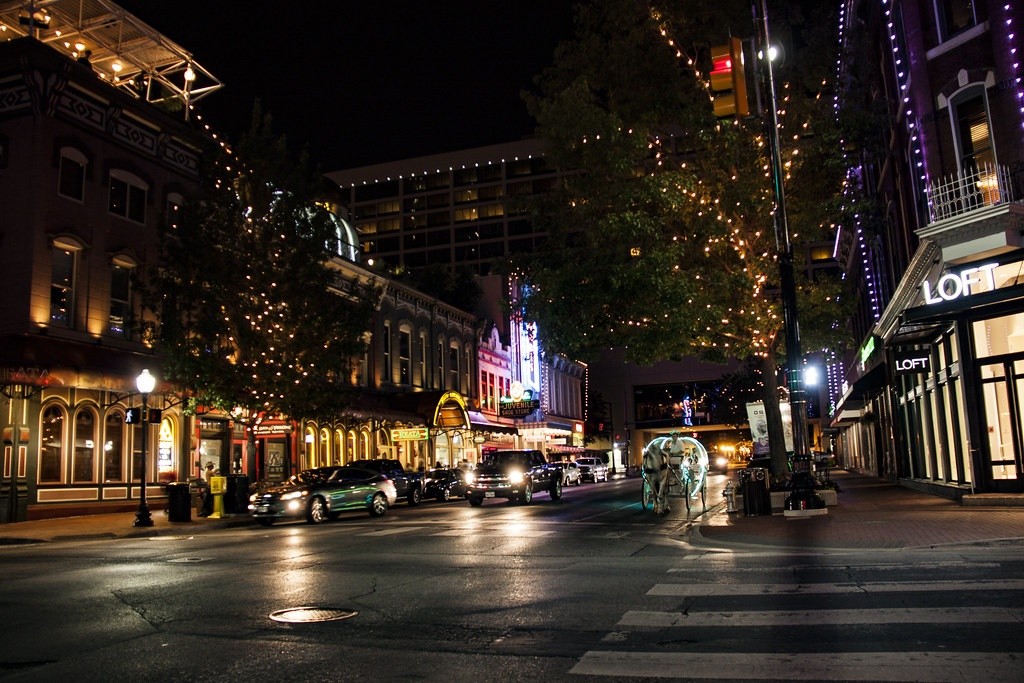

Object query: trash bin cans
[167,481,191,522]
[738,467,772,517]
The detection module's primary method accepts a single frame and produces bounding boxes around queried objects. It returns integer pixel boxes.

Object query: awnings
[547,443,585,455]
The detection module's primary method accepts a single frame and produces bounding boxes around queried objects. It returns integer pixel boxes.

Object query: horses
[642,442,671,515]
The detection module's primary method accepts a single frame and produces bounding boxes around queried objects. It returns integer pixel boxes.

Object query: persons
[404,463,411,470]
[435,462,441,467]
[669,434,686,472]
[459,459,468,470]
[203,461,221,516]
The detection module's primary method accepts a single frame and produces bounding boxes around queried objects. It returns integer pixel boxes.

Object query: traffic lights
[708,34,751,119]
[148,408,162,424]
[124,406,141,425]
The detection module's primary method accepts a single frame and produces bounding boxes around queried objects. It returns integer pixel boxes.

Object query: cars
[247,464,398,528]
[550,461,583,487]
[423,466,468,504]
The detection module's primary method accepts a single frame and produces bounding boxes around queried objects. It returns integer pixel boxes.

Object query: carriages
[641,437,709,516]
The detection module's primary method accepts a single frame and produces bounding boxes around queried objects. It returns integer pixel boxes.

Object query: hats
[205,461,216,468]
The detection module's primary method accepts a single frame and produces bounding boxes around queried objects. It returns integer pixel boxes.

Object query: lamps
[96,337,102,345]
[39,327,48,336]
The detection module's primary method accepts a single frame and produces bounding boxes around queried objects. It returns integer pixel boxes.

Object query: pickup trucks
[466,447,565,507]
[342,458,426,507]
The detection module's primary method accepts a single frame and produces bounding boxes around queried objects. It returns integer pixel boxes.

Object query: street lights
[132,367,155,527]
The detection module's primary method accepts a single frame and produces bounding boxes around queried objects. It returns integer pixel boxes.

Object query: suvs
[573,456,609,484]
